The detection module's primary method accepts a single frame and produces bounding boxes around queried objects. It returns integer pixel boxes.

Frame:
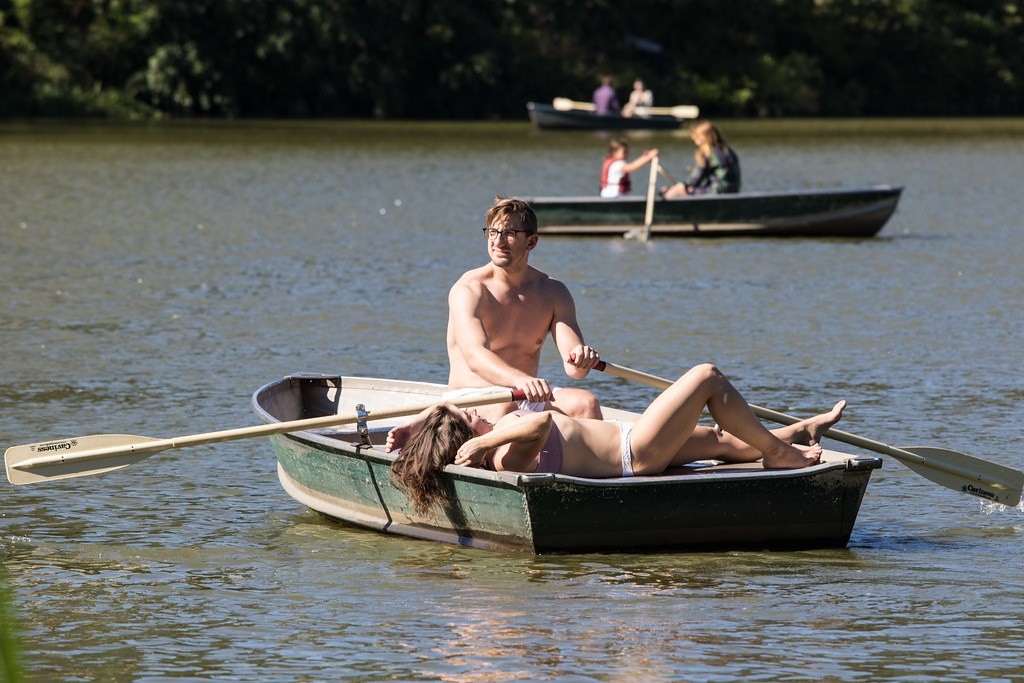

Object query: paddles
[4,386,553,489]
[552,96,700,120]
[570,350,1023,509]
[642,155,659,240]
[657,163,675,185]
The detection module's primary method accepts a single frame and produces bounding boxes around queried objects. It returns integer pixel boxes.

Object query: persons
[594,75,625,116]
[441,193,603,422]
[621,79,653,119]
[385,364,846,523]
[659,122,742,198]
[598,138,659,198]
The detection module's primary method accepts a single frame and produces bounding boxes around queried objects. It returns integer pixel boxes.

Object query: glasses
[483,226,529,240]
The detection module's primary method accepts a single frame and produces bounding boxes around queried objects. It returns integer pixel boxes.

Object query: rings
[458,453,461,459]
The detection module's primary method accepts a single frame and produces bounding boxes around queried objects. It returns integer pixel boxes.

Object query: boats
[525,100,683,132]
[515,183,909,236]
[248,368,889,558]
